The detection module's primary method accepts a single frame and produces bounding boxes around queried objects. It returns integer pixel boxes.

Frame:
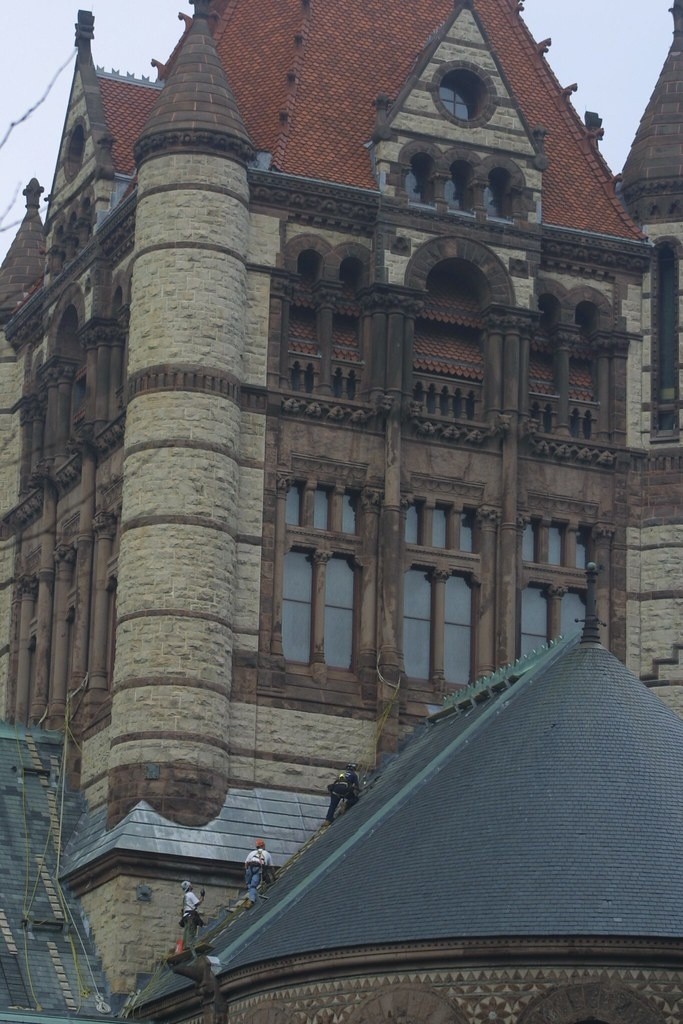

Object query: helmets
[346,763,356,770]
[256,839,265,847]
[181,881,190,892]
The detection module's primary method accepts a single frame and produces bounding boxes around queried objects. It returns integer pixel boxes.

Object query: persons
[321,764,360,826]
[182,881,205,951]
[242,839,278,909]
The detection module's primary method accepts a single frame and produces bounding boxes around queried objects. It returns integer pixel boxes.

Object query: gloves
[200,888,205,896]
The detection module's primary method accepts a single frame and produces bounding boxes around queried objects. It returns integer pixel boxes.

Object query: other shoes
[243,903,251,908]
[321,821,329,827]
[340,803,346,814]
